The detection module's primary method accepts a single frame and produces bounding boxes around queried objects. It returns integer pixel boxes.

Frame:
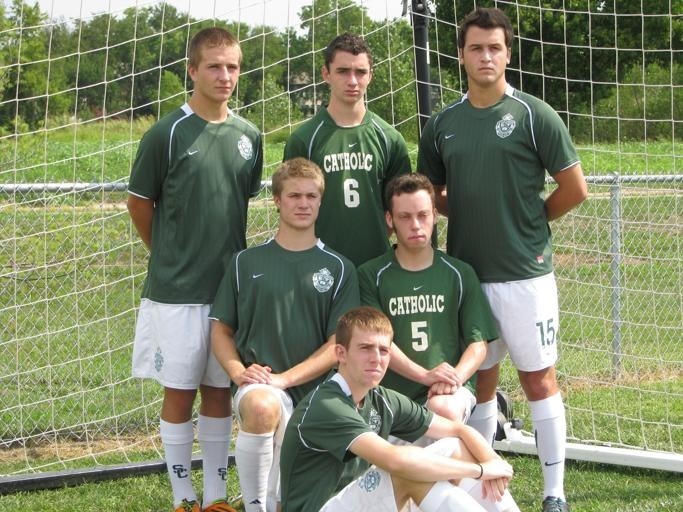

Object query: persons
[280,306,521,512]
[282,29,412,268]
[417,6,588,511]
[356,170,501,471]
[205,156,361,512]
[125,27,264,510]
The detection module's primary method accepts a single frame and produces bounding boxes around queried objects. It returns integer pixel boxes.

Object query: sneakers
[174,499,236,511]
[542,497,568,512]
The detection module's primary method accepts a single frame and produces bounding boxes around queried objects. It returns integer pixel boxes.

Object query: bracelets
[474,463,483,480]
